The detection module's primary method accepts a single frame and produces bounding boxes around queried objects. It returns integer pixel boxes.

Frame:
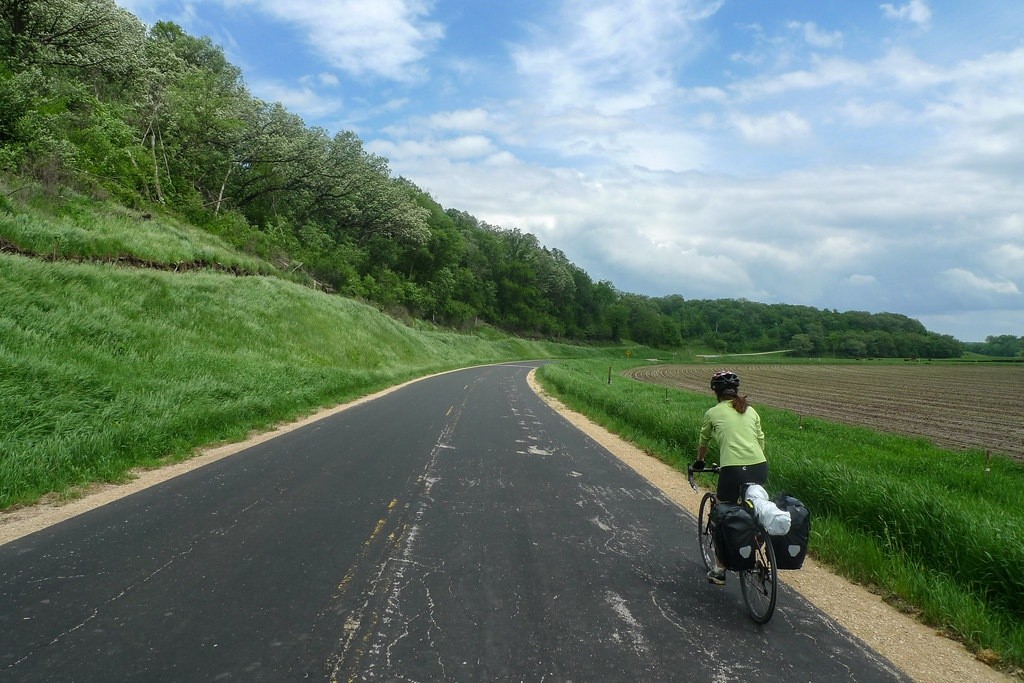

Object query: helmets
[711,371,739,390]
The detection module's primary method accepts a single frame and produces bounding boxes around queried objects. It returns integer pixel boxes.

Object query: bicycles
[685,461,778,626]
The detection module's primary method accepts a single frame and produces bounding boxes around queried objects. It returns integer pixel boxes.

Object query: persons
[692,370,768,585]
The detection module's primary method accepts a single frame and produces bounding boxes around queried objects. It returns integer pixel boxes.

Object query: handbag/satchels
[707,501,759,572]
[766,495,810,570]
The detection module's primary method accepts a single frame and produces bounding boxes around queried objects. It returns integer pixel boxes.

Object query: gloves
[692,459,705,470]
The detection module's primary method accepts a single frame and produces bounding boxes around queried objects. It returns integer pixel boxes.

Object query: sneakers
[706,567,726,584]
